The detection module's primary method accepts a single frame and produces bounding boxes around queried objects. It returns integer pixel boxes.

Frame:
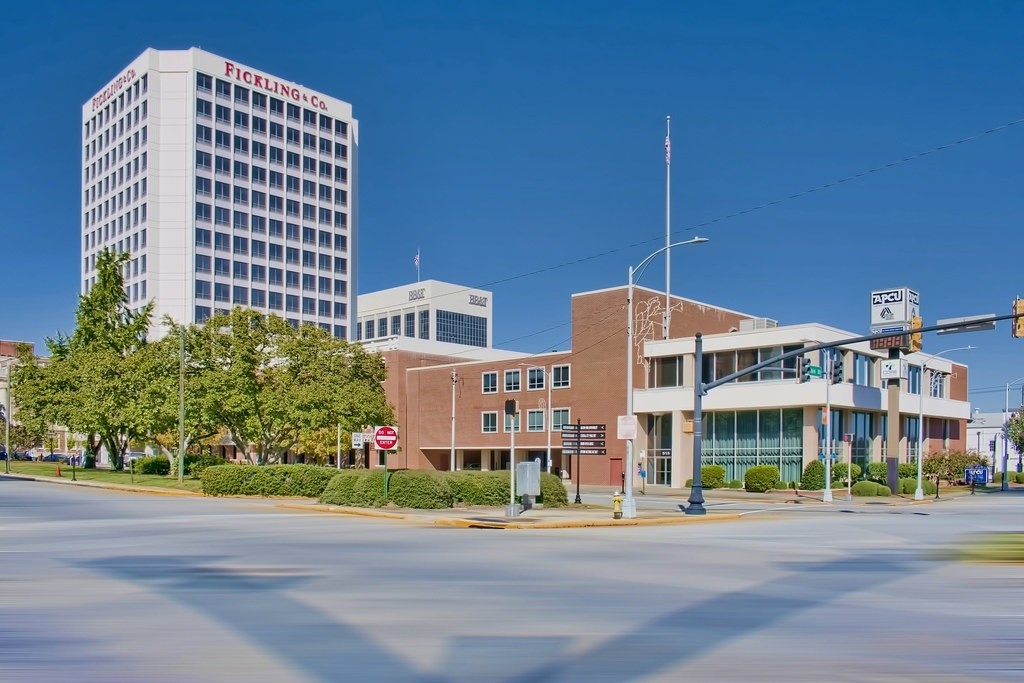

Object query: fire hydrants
[612,490,624,519]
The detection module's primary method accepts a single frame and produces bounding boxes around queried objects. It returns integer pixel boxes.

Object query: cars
[15,453,32,461]
[123,452,145,470]
[44,454,65,462]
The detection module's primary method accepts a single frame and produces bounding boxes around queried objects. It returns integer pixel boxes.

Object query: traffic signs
[351,432,363,449]
[560,424,606,455]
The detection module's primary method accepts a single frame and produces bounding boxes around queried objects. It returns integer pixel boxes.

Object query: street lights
[516,362,552,473]
[798,338,833,501]
[624,235,710,518]
[916,346,977,501]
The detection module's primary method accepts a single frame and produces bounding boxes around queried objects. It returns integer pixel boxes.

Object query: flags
[414,252,418,266]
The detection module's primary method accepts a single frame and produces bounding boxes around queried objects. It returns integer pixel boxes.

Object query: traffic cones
[55,467,62,477]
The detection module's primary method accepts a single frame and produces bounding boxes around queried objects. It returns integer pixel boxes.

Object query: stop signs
[376,428,398,449]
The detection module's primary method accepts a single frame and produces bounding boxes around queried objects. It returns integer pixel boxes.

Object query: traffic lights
[831,361,843,385]
[800,358,811,382]
